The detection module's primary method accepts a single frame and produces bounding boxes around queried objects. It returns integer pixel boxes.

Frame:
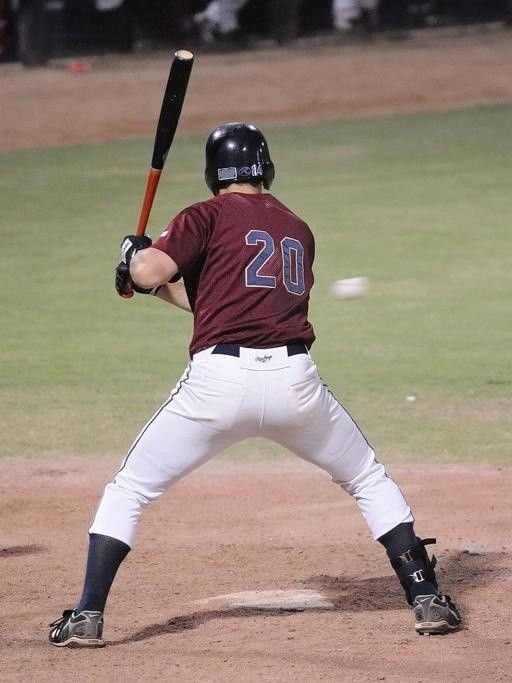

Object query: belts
[210,343,311,357]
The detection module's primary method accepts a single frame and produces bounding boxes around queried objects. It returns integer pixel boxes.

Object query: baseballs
[339,278,366,300]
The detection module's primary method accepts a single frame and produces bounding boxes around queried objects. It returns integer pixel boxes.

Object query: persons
[44,119,464,649]
[1,1,512,70]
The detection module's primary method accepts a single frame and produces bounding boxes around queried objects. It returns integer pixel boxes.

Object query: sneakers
[48,607,106,649]
[411,592,462,635]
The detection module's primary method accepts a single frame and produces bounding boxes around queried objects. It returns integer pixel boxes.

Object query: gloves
[120,232,152,265]
[114,261,163,298]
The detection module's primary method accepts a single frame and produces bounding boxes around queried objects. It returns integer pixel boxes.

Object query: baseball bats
[120,49,193,298]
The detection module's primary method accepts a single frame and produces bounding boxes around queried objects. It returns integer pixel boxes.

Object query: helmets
[204,121,276,196]
